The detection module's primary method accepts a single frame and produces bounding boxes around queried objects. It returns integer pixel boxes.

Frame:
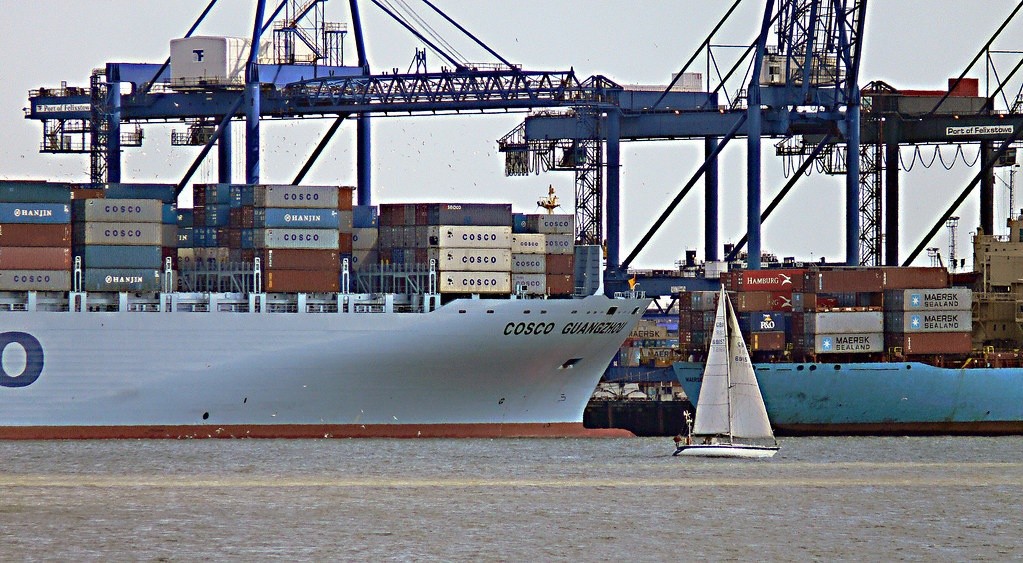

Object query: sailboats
[672,282,782,458]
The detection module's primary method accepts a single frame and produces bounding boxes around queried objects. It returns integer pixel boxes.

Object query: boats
[671,209,1023,436]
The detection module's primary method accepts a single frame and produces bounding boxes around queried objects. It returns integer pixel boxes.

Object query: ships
[0,180,654,440]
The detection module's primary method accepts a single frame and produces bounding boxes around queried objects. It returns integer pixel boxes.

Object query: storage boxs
[610,91,719,112]
[860,77,994,116]
[0,182,1023,401]
[170,37,362,94]
